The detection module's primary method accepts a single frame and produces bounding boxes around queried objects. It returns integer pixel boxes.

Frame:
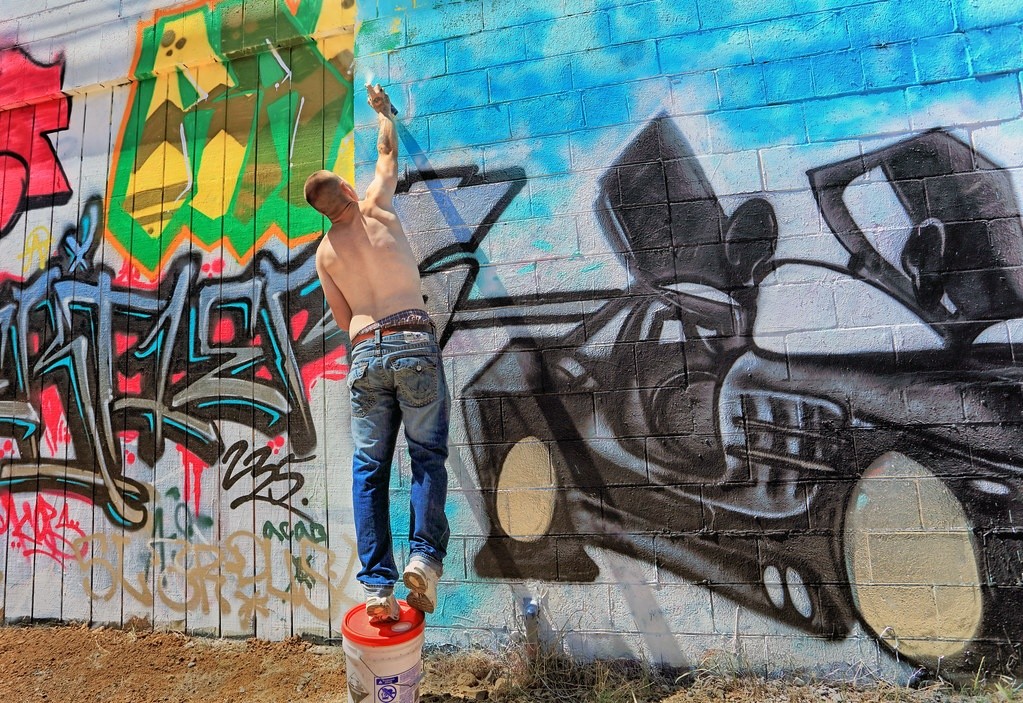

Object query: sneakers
[366,593,402,623]
[402,558,439,614]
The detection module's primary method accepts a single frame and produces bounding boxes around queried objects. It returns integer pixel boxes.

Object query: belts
[351,324,435,351]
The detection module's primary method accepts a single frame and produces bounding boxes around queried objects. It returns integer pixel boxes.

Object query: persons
[304,83,452,625]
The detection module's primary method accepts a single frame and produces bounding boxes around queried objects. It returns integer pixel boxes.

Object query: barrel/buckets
[342,599,426,703]
